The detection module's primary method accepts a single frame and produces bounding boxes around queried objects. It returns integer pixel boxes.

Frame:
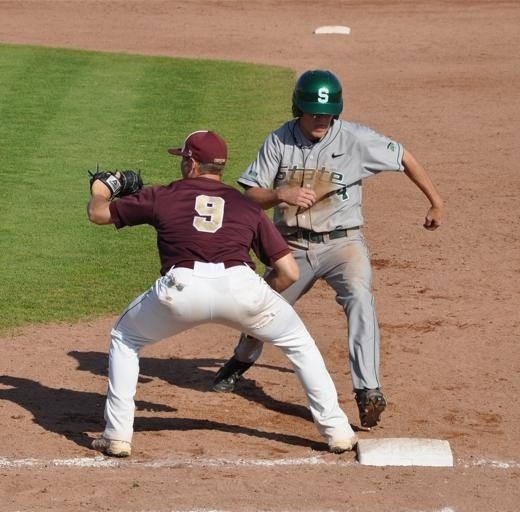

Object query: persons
[88,130,358,458]
[211,70,443,427]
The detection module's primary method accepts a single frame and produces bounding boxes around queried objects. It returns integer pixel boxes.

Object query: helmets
[291,69,343,121]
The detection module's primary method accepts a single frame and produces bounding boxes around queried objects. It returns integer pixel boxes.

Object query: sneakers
[327,435,358,454]
[91,436,132,458]
[211,358,254,391]
[354,387,387,427]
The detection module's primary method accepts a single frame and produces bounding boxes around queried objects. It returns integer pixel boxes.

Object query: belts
[175,260,245,270]
[301,228,347,242]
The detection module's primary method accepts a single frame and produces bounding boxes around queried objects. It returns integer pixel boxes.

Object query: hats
[168,130,228,165]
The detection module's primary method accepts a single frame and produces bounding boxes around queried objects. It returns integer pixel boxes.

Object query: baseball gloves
[90,168,142,200]
